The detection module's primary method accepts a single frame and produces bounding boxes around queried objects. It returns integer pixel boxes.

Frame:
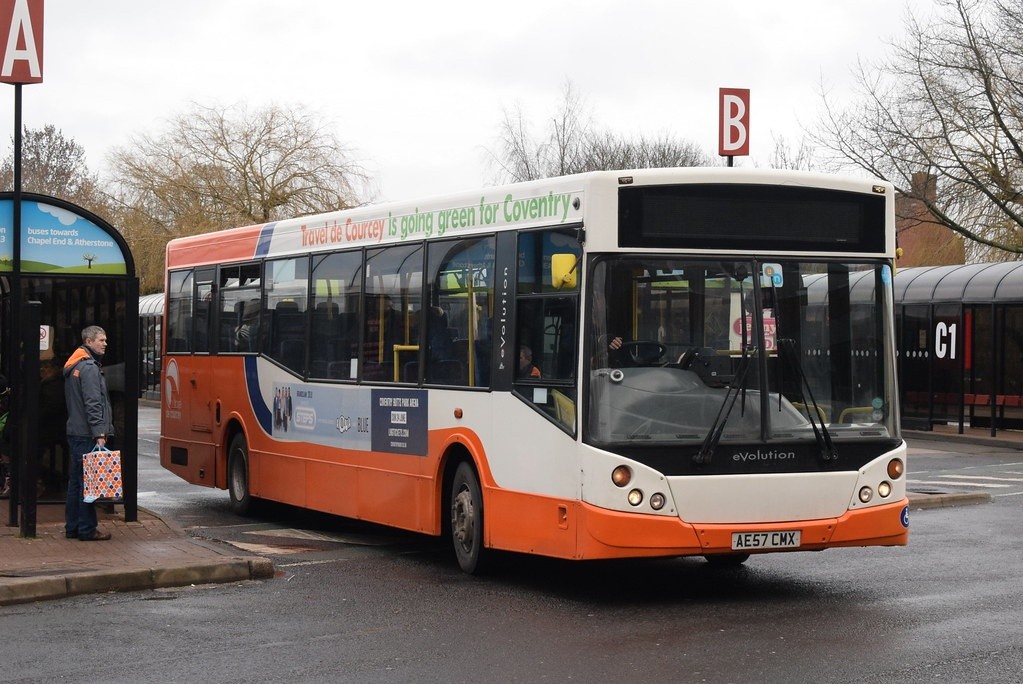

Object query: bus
[159,166,913,572]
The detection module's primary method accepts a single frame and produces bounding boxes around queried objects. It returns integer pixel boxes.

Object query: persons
[235,295,455,383]
[0,360,65,500]
[520,347,540,378]
[64,326,114,540]
[558,295,622,367]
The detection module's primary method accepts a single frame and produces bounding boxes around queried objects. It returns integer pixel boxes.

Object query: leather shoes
[79,529,111,540]
[66,527,82,539]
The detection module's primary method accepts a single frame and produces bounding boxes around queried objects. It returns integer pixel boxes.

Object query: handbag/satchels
[82,444,124,503]
